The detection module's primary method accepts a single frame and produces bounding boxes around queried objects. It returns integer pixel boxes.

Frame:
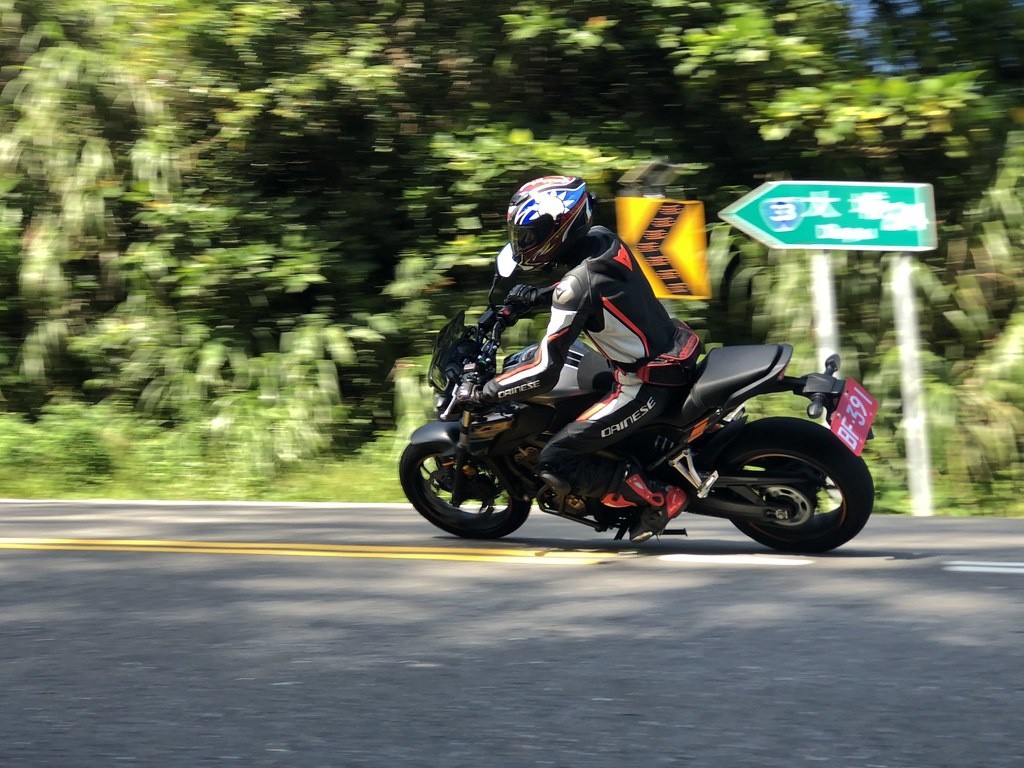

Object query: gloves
[504,283,540,320]
[455,382,485,408]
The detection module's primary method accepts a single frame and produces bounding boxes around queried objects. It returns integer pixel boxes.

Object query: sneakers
[599,453,688,543]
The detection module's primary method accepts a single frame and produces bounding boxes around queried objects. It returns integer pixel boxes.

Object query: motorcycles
[398,243,878,555]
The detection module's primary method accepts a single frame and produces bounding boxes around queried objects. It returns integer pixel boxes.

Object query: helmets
[507,174,593,268]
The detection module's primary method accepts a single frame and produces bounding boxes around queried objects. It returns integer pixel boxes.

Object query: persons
[457,176,707,542]
